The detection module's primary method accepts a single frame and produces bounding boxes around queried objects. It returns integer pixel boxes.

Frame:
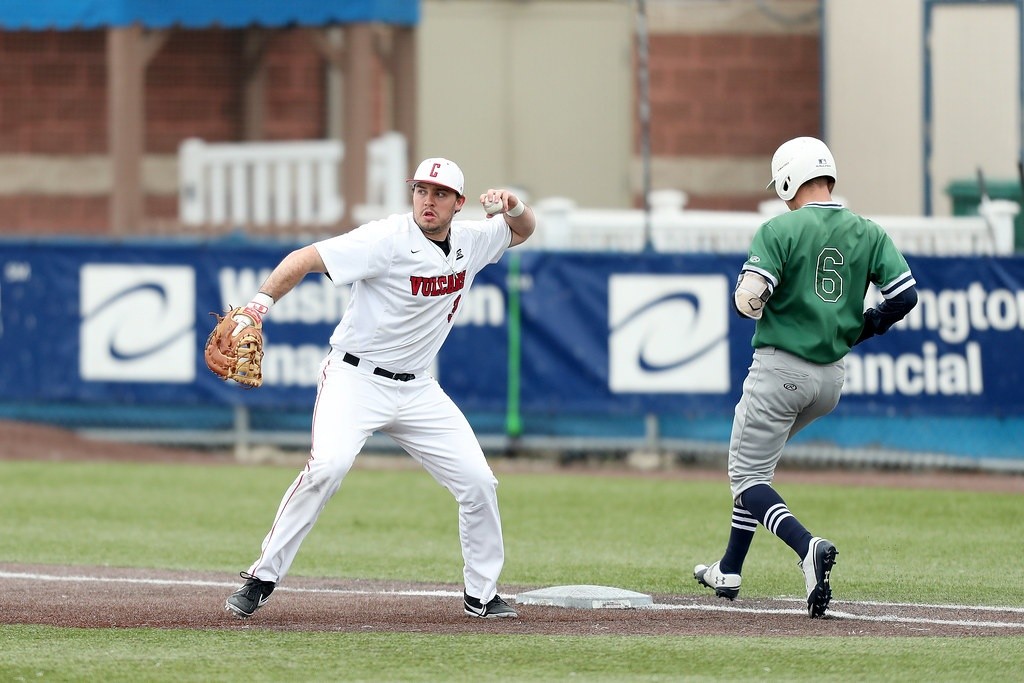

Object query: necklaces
[426,230,458,279]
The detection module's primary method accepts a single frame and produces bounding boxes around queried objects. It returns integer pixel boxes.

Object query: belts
[343,353,415,381]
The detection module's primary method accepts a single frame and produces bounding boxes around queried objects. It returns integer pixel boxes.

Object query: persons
[222,157,536,618]
[691,134,918,620]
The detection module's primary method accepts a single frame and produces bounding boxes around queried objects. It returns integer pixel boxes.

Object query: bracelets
[505,196,525,217]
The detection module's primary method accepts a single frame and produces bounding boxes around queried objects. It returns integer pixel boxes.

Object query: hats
[406,157,464,196]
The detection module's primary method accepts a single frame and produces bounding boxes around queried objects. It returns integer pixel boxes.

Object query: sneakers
[693,561,742,601]
[464,588,518,619]
[224,571,275,620]
[797,536,839,618]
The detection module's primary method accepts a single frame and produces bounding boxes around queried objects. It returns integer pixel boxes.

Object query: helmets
[766,137,837,200]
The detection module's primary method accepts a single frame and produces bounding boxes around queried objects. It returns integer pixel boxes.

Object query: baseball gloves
[204,301,266,391]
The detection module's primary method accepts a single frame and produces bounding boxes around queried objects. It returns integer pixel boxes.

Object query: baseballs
[482,195,504,215]
